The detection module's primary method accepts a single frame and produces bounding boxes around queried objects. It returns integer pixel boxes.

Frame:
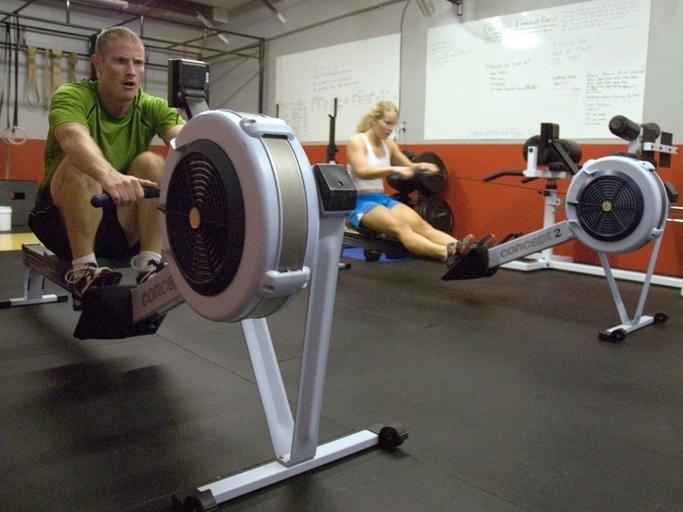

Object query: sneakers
[64,262,113,310]
[445,234,496,269]
[129,253,165,285]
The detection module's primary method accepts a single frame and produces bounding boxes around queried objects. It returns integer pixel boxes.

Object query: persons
[344,99,496,269]
[27,27,187,310]
